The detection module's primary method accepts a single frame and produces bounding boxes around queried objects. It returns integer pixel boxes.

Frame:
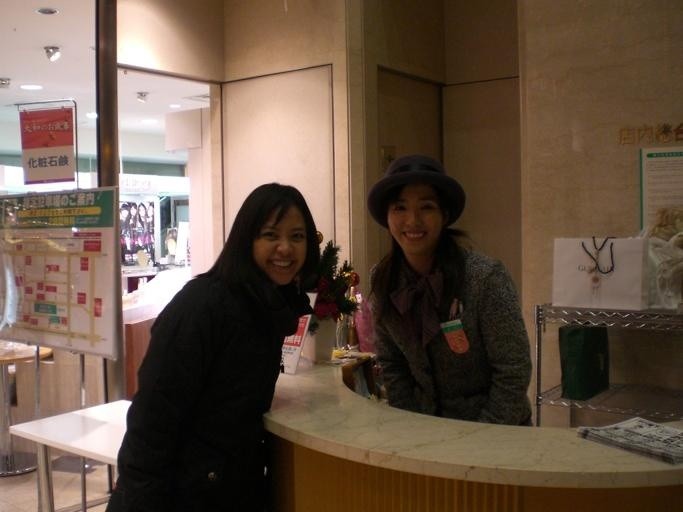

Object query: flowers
[303,231,361,333]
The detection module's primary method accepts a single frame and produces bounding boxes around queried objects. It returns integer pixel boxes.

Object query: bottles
[354,291,376,354]
[336,286,359,350]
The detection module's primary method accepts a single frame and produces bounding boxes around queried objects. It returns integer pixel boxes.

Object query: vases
[299,319,337,362]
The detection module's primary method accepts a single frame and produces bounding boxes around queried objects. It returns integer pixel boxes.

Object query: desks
[9,399,132,512]
[0,339,52,477]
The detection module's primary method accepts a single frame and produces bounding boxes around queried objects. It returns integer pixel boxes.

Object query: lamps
[136,92,147,103]
[44,47,61,61]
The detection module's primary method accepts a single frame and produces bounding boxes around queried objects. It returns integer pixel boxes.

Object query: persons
[117,201,155,266]
[104,182,323,511]
[366,155,533,427]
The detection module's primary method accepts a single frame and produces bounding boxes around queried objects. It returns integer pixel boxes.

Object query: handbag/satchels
[559,325,610,399]
[552,236,649,311]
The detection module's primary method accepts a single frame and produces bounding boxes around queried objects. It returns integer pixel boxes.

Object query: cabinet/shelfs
[535,302,682,427]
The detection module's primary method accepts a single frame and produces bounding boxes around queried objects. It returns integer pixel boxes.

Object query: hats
[368,154,465,230]
